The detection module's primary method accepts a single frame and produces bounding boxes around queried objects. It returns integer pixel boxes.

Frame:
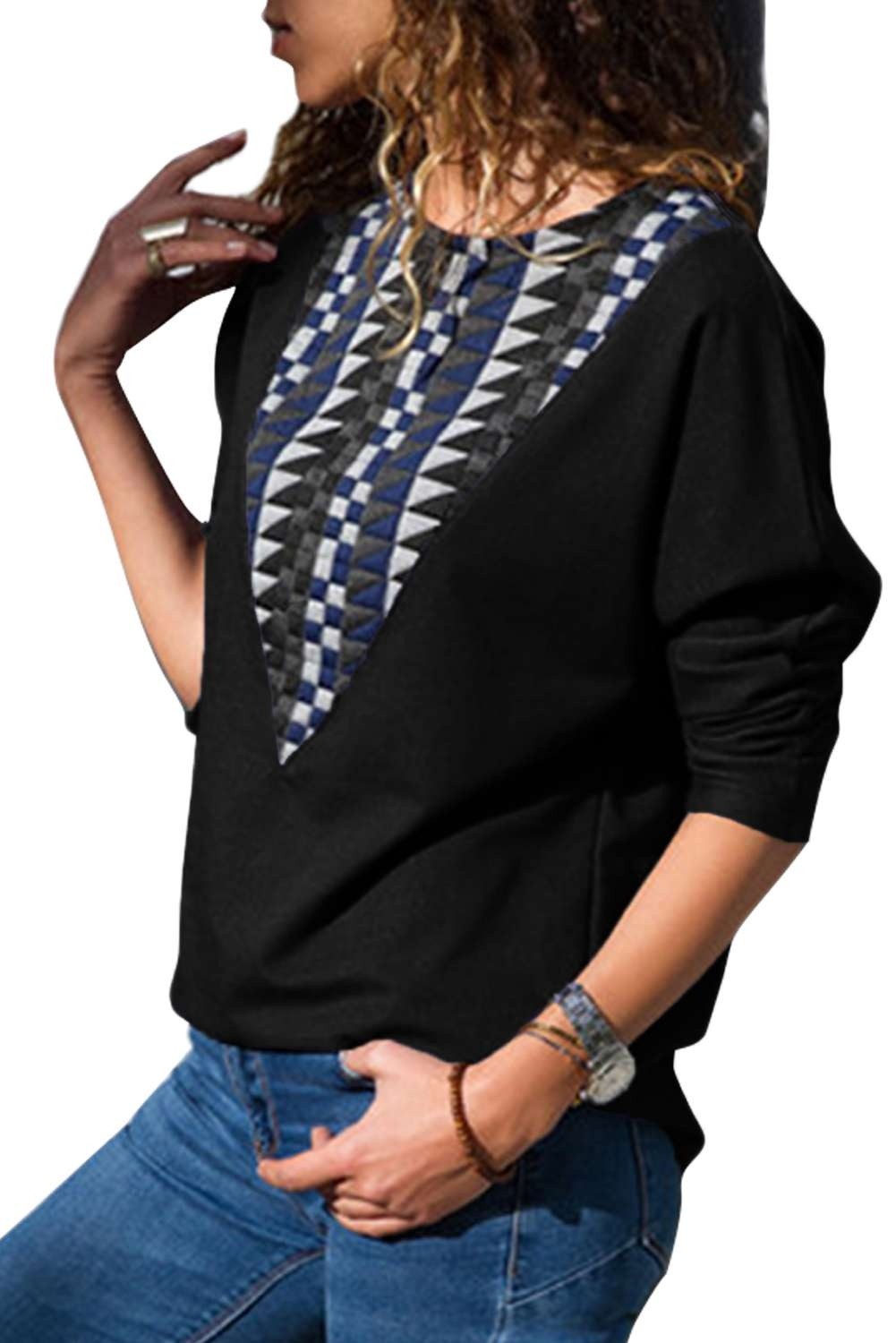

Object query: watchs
[552,979,637,1102]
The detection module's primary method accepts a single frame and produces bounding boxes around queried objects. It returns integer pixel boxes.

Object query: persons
[0,0,883,1343]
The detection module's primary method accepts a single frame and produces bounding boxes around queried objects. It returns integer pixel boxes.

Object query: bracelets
[448,1061,519,1184]
[520,1021,592,1079]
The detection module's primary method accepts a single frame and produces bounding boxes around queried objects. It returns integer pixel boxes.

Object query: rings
[146,241,172,273]
[136,214,191,241]
